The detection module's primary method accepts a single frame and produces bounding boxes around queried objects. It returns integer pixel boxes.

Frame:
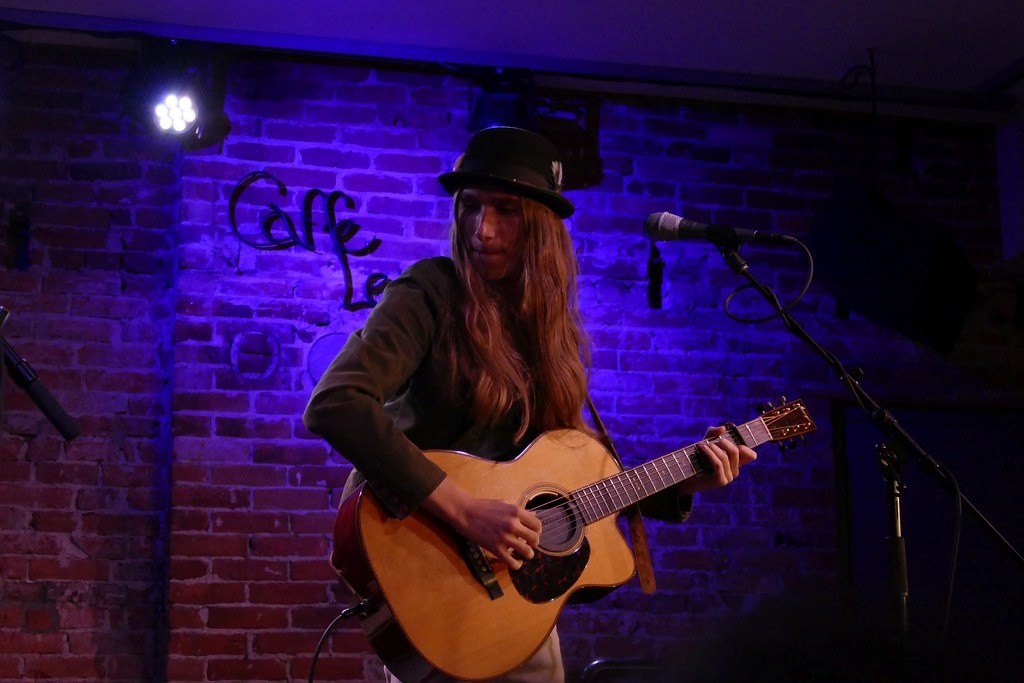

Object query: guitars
[329,391,821,683]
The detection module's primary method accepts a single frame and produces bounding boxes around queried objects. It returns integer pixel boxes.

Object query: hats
[438,125,575,217]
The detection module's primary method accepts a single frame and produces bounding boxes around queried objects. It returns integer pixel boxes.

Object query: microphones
[645,212,795,249]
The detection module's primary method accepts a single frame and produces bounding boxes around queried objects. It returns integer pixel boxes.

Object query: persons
[307,124,759,681]
